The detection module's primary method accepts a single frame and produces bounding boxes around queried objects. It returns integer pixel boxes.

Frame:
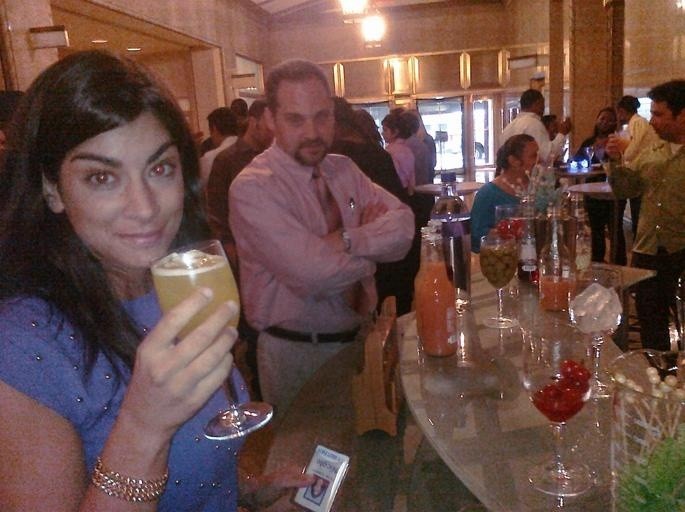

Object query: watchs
[336,227,352,252]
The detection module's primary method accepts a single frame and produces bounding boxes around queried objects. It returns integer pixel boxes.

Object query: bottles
[441,171,456,196]
[414,226,428,346]
[421,218,460,356]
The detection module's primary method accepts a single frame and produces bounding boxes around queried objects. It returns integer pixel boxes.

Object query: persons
[498,90,574,167]
[613,94,657,238]
[599,78,684,384]
[328,96,422,315]
[353,106,382,156]
[193,106,238,193]
[302,475,335,508]
[544,113,561,140]
[0,48,252,511]
[231,97,250,136]
[228,62,415,421]
[574,108,628,268]
[206,100,274,227]
[384,100,440,220]
[0,91,19,152]
[469,133,544,259]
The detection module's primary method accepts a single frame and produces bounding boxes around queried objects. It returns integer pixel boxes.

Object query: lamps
[340,2,369,25]
[361,7,387,49]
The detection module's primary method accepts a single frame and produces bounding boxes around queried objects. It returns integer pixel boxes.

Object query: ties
[313,166,372,319]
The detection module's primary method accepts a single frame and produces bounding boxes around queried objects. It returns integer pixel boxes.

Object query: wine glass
[586,147,595,169]
[614,130,631,168]
[495,204,529,297]
[519,322,596,495]
[479,233,520,331]
[566,264,624,400]
[149,239,273,441]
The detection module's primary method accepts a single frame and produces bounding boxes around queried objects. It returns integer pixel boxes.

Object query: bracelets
[89,454,170,503]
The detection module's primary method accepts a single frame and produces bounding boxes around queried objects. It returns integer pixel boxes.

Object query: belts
[263,326,359,344]
